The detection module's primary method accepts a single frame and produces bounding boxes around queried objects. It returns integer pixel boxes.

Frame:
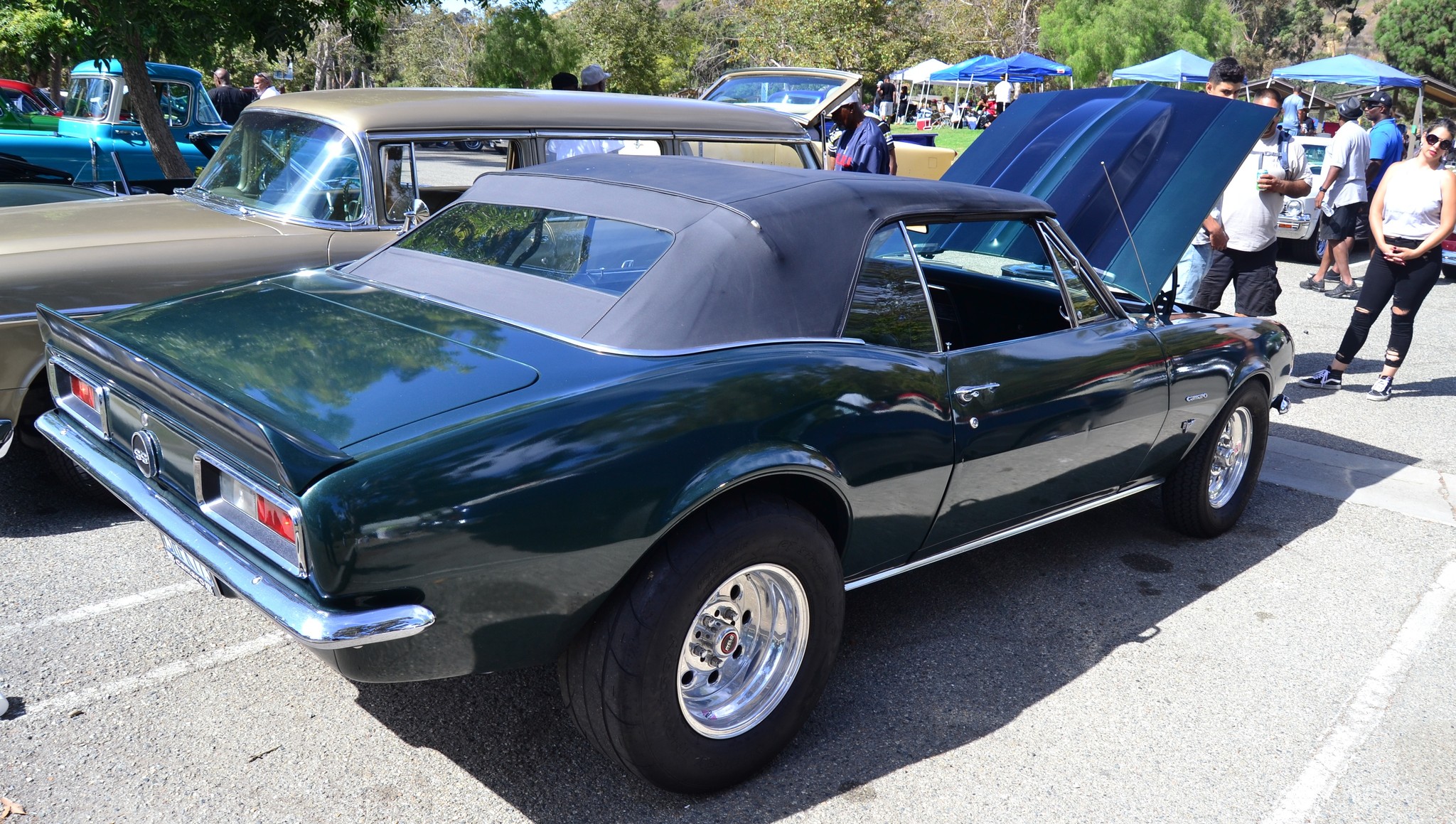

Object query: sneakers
[1298,365,1342,390]
[1367,374,1394,401]
[1325,279,1360,298]
[1300,274,1325,292]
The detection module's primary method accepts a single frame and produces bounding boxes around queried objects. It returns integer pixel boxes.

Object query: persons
[1163,58,1245,306]
[1281,84,1315,136]
[250,72,282,106]
[1308,91,1403,283]
[278,85,286,94]
[300,84,310,92]
[906,94,997,128]
[824,85,890,176]
[894,86,908,124]
[1300,94,1371,301]
[1298,104,1315,136]
[1298,115,1456,402]
[1192,89,1314,317]
[878,75,896,127]
[823,84,897,176]
[873,81,884,116]
[549,65,625,164]
[206,67,251,126]
[993,73,1014,116]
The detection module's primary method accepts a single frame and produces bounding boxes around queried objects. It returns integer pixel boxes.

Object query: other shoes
[1312,271,1341,283]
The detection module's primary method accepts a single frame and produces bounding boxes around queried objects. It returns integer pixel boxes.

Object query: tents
[1109,49,1253,103]
[1267,54,1424,156]
[922,54,1044,129]
[890,57,988,130]
[957,51,1073,129]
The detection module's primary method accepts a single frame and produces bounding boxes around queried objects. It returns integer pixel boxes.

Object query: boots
[1442,159,1447,166]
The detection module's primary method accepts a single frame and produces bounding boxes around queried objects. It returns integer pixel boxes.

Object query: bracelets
[1319,186,1327,192]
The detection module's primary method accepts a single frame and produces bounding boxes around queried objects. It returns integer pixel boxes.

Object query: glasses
[212,70,219,80]
[1365,103,1382,109]
[1276,107,1282,117]
[1425,132,1452,150]
[254,72,268,81]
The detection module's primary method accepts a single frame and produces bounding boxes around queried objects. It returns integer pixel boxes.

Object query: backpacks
[978,113,990,128]
[917,108,933,118]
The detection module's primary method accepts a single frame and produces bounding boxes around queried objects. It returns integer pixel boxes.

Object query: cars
[35,86,1298,795]
[618,68,959,232]
[1275,136,1369,265]
[1440,224,1456,280]
[0,86,827,499]
[0,59,358,207]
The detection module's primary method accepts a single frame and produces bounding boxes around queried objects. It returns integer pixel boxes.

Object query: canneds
[1257,169,1269,191]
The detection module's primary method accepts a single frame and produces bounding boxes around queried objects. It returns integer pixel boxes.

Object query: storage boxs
[916,117,933,131]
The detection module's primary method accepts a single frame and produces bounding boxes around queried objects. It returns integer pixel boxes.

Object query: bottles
[1320,201,1335,217]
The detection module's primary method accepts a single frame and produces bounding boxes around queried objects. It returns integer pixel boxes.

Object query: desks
[957,106,970,128]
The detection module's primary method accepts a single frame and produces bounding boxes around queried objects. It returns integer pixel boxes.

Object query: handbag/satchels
[950,98,960,123]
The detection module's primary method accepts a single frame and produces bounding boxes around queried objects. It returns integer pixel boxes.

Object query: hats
[941,95,950,101]
[581,64,611,86]
[877,81,883,86]
[902,86,908,90]
[882,75,890,80]
[1303,105,1310,113]
[929,99,937,103]
[1334,96,1363,119]
[1362,90,1393,109]
[826,86,859,114]
[551,72,578,89]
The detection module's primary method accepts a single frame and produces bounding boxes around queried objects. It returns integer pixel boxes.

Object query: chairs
[410,186,468,217]
[914,104,953,131]
